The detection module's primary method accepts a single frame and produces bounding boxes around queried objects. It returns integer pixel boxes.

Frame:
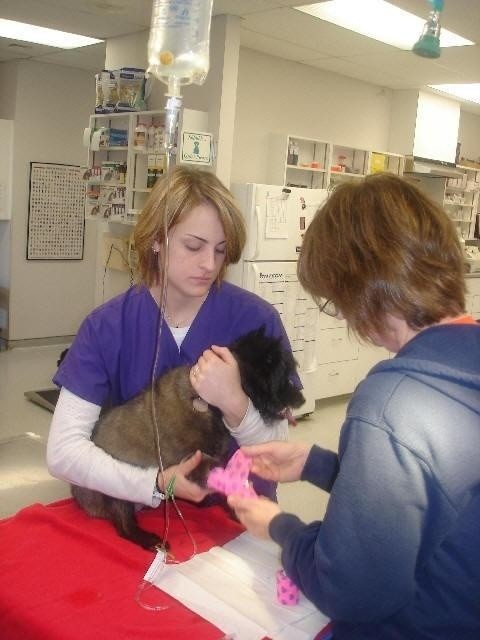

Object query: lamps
[411,0,443,58]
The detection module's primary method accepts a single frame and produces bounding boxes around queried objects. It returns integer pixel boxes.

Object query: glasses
[311,292,342,317]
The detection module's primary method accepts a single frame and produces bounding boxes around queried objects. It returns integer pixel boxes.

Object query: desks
[0,497,332,639]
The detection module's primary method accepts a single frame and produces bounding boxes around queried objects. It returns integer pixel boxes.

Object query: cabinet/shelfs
[317,277,479,400]
[265,133,405,190]
[82,107,208,227]
[416,164,480,246]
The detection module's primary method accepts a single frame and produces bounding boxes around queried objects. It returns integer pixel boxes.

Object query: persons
[46,164,302,511]
[228,172,479,639]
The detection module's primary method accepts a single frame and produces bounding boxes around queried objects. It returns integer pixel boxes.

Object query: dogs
[69,324,307,554]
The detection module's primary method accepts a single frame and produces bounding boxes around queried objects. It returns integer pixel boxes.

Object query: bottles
[133,123,164,152]
[339,155,347,167]
[105,187,126,215]
[119,160,126,185]
[287,141,298,166]
